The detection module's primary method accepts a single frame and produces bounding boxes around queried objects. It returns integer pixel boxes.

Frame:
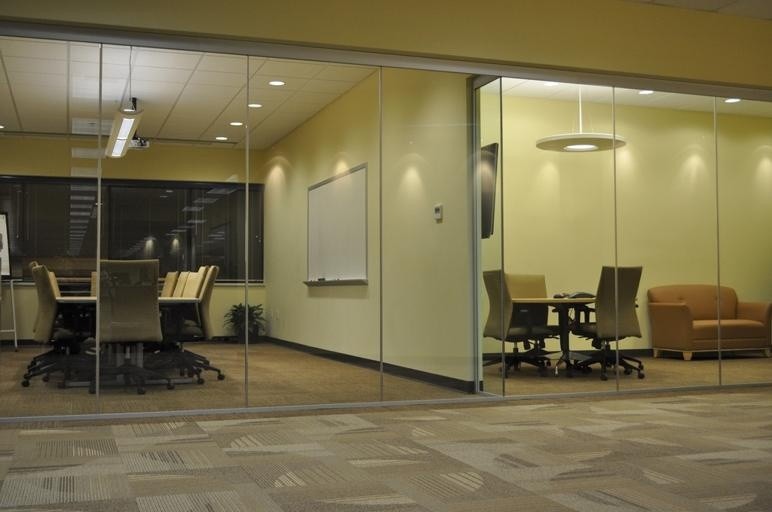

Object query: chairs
[21,258,226,396]
[480,265,646,383]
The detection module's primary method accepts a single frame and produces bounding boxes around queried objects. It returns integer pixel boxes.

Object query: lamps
[535,84,627,155]
[104,45,147,159]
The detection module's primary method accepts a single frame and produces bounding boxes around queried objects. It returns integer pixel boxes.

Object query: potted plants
[222,302,270,345]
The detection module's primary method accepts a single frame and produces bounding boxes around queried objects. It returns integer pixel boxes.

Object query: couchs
[648,283,772,362]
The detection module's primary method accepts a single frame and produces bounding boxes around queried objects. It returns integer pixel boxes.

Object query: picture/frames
[0,209,14,281]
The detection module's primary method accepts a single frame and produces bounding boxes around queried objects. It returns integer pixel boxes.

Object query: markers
[317,278,325,280]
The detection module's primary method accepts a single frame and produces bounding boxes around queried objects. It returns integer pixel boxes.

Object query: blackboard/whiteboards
[301,161,370,287]
